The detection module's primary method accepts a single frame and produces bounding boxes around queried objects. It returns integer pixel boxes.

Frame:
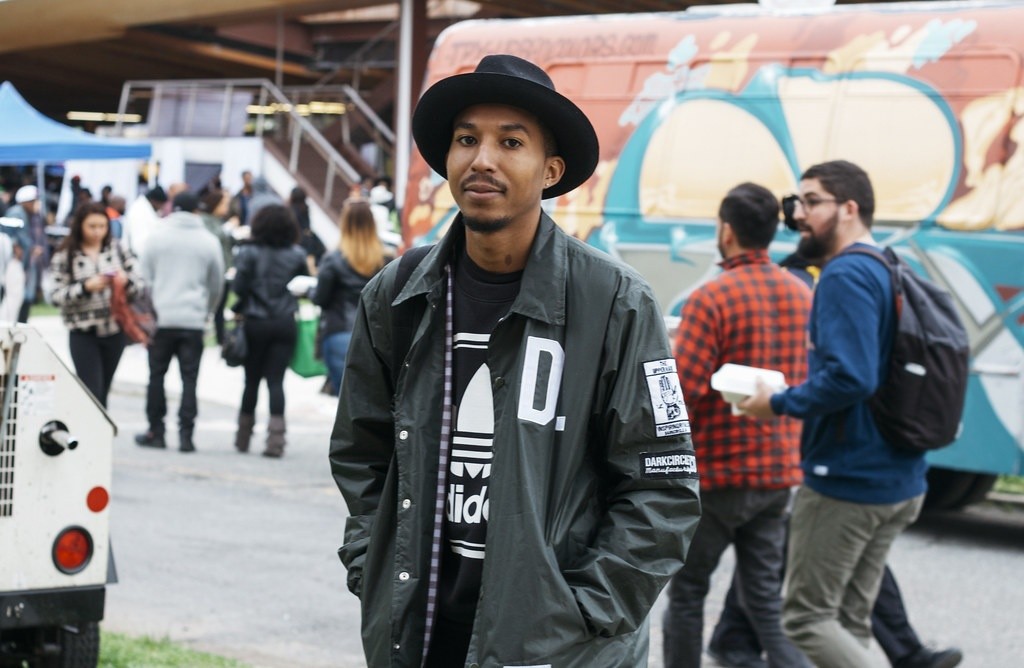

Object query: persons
[314,203,396,397]
[735,158,929,668]
[0,165,399,302]
[41,202,146,412]
[232,203,311,459]
[133,192,224,453]
[707,195,963,668]
[328,55,698,668]
[662,182,821,668]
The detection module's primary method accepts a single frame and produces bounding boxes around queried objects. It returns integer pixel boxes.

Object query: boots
[260,416,286,457]
[234,413,256,451]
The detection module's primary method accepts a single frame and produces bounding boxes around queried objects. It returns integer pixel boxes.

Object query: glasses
[800,189,833,206]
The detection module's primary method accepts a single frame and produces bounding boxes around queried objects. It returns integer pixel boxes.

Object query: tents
[0,80,152,265]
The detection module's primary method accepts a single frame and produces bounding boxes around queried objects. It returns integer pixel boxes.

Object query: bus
[402,0,1023,528]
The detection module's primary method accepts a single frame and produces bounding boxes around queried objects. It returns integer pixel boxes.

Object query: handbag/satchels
[110,288,159,342]
[221,328,246,368]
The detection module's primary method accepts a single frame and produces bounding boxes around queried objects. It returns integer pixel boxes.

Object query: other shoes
[180,437,194,450]
[135,433,166,448]
[893,647,962,668]
[710,646,769,668]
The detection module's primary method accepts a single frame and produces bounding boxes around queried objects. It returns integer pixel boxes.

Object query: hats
[15,184,37,203]
[173,193,197,212]
[370,186,395,203]
[411,54,600,200]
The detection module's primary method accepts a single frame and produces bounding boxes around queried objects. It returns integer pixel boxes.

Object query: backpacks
[838,248,971,453]
